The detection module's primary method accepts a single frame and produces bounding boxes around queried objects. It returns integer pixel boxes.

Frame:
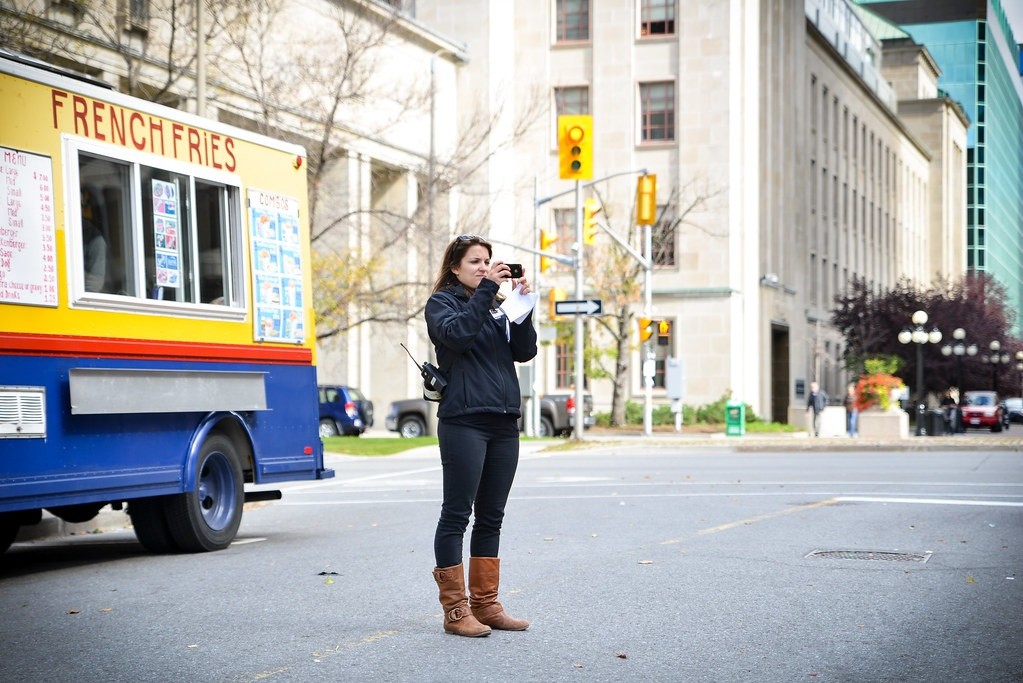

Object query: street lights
[942,326,979,391]
[980,335,1011,400]
[898,310,942,432]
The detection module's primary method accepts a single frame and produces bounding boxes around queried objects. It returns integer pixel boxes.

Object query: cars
[961,390,1023,432]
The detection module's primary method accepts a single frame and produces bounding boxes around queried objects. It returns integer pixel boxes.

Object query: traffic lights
[583,197,602,246]
[539,230,557,272]
[657,320,671,335]
[556,115,595,180]
[639,317,653,340]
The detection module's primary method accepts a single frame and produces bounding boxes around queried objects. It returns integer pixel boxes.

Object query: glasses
[452,235,486,254]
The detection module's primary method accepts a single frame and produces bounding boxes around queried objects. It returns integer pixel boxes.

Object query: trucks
[0,50,336,553]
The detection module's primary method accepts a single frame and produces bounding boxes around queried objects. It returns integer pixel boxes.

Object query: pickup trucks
[385,393,596,437]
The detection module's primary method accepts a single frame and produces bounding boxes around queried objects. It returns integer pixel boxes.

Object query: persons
[806,382,827,437]
[81,187,108,292]
[843,383,859,438]
[425,235,538,636]
[940,390,956,416]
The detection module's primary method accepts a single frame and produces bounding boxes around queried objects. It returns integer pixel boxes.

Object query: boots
[468,557,530,631]
[432,560,492,637]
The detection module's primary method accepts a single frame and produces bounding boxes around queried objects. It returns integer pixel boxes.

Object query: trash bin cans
[927,409,945,435]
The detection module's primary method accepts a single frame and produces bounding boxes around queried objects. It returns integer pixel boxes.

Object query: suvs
[318,383,374,438]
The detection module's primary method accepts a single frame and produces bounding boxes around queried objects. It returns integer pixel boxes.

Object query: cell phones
[498,264,522,278]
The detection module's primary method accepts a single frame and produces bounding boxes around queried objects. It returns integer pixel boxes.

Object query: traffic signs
[555,298,603,317]
